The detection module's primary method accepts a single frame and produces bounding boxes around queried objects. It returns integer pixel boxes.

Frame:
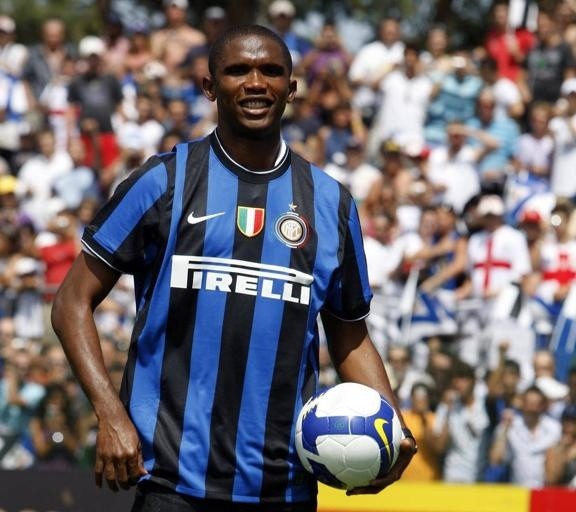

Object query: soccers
[294,383,403,492]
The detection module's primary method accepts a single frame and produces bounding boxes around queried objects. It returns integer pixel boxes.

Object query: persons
[49,23,421,511]
[2,0,576,492]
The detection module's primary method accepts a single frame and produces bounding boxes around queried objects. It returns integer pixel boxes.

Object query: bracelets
[402,428,416,444]
